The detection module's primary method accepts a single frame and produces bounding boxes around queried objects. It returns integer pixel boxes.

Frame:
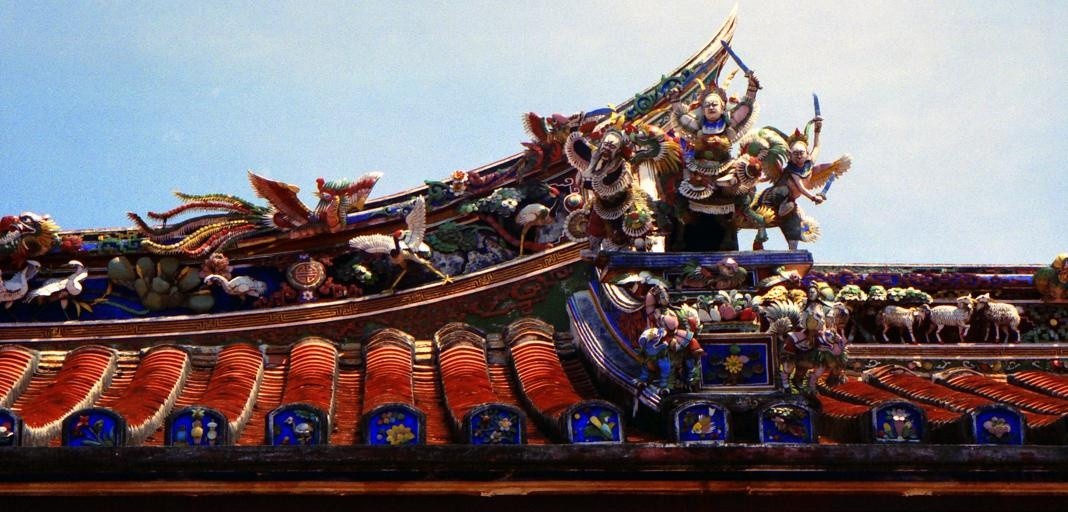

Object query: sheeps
[879,293,1033,343]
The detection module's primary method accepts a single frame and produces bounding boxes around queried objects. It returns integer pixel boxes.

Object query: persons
[668,75,760,253]
[752,120,824,251]
[565,112,665,260]
[643,286,704,391]
[780,274,849,391]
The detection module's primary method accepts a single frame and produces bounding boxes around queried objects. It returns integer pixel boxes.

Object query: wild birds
[348,194,461,294]
[513,182,563,259]
